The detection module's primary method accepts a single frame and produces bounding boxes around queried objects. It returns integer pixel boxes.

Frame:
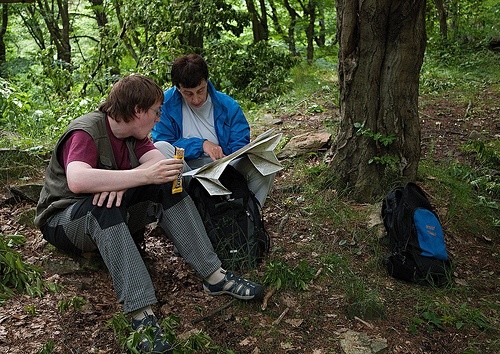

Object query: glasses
[142,106,162,118]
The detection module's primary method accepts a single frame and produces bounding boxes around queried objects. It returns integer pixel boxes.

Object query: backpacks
[381,182,450,281]
[190,164,270,270]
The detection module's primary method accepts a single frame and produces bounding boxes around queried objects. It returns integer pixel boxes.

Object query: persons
[33,74,261,354]
[152,53,271,259]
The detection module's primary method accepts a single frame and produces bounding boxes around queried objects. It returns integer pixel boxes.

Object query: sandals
[203,270,257,299]
[132,311,173,354]
